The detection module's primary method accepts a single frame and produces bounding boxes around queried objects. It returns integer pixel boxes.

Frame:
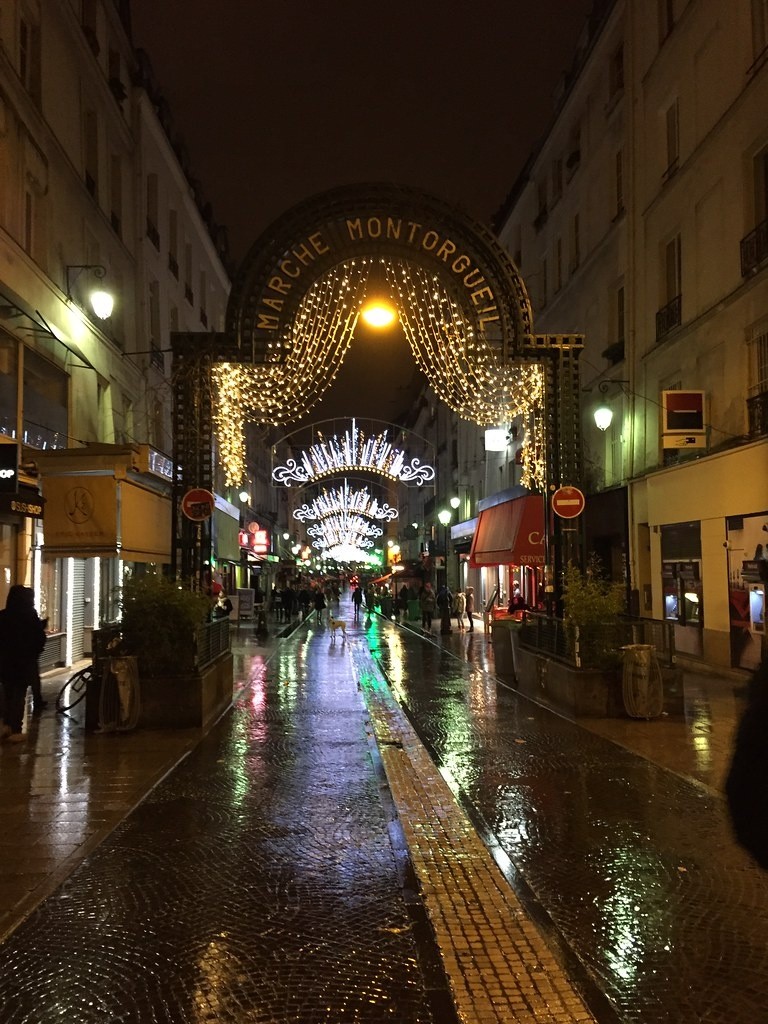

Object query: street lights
[437,510,452,591]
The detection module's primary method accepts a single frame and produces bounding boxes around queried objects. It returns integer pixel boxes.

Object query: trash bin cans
[257,610,269,634]
[380,598,393,617]
[622,644,663,720]
[100,657,141,730]
[490,619,516,677]
[407,600,422,622]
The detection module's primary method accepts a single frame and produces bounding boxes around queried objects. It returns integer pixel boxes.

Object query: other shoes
[10,734,27,742]
[36,702,49,708]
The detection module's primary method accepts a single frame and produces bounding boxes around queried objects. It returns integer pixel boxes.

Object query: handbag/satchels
[323,604,326,609]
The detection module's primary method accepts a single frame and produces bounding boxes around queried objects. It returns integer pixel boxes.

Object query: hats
[425,583,431,589]
[467,587,474,593]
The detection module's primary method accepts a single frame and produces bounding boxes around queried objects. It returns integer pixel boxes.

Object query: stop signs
[553,485,587,519]
[182,489,215,520]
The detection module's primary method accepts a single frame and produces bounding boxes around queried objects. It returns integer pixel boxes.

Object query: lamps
[593,379,631,431]
[437,483,470,524]
[66,265,113,319]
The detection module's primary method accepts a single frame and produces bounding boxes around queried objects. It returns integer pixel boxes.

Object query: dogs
[327,616,346,633]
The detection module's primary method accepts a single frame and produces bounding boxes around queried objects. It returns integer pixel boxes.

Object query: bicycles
[54,665,95,713]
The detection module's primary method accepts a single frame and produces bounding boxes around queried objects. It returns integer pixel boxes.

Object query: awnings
[467,496,553,570]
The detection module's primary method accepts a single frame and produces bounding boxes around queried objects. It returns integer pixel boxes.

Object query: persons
[20,588,48,708]
[454,588,466,629]
[513,580,520,597]
[465,586,475,633]
[419,582,436,629]
[253,585,343,624]
[0,585,34,743]
[436,585,454,627]
[364,585,416,612]
[351,588,363,615]
[214,589,233,615]
